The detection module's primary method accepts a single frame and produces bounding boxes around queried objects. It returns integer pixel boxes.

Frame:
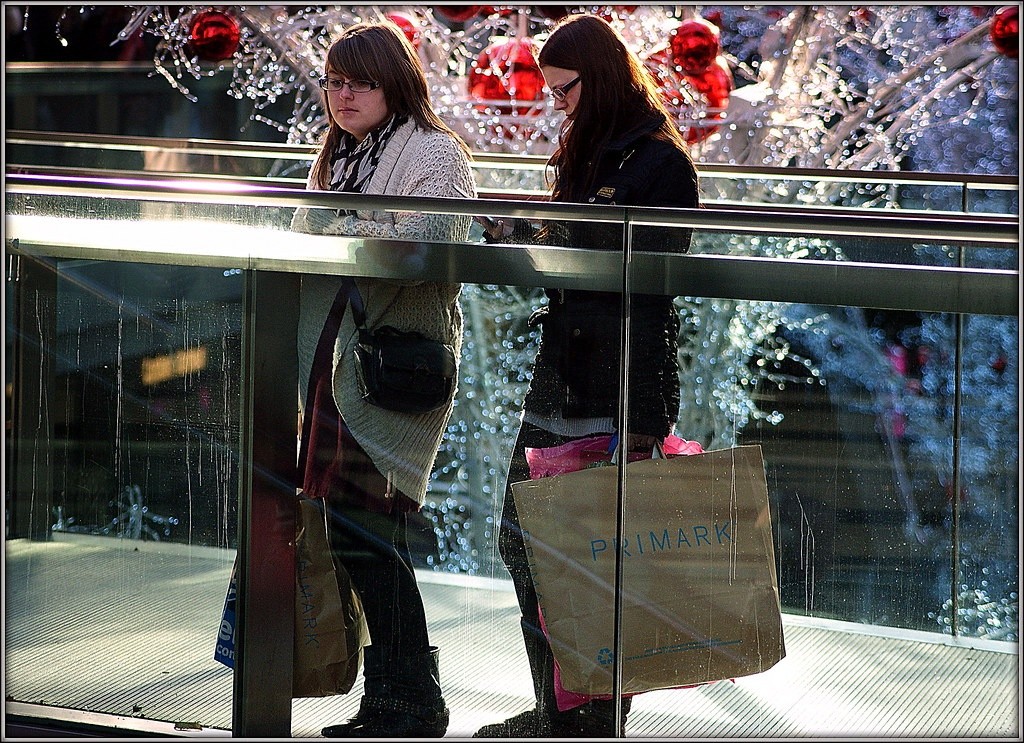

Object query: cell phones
[452,182,498,226]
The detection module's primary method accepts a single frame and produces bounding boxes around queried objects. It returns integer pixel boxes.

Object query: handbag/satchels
[510,430,787,713]
[351,325,458,416]
[293,496,371,699]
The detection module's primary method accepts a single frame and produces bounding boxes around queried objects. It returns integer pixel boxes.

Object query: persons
[490,12,699,738]
[284,20,480,739]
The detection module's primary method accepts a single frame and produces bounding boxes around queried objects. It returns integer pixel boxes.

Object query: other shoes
[539,694,632,738]
[472,695,549,738]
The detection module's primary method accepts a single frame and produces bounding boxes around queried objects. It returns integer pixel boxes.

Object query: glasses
[549,76,583,101]
[319,73,381,94]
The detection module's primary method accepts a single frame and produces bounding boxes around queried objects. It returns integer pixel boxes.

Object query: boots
[321,644,376,739]
[351,647,449,737]
